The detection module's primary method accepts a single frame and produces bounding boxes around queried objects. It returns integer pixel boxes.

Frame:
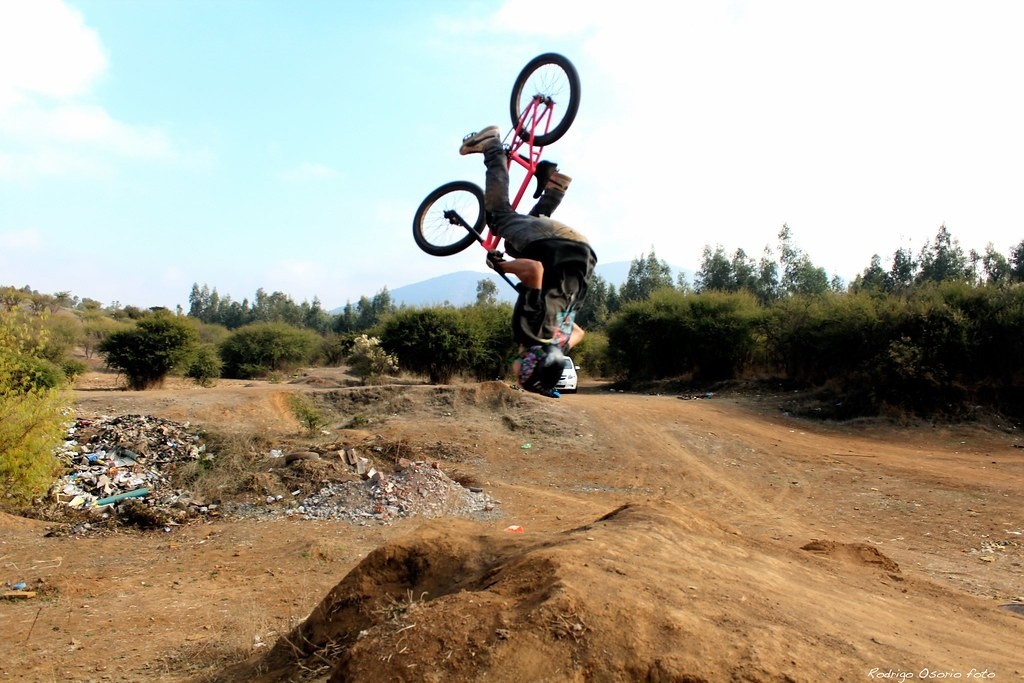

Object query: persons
[459,125,601,395]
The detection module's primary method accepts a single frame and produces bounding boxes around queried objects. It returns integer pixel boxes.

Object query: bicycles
[412,53,581,287]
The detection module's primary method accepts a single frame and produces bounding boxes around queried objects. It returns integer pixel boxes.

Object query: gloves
[485,249,506,272]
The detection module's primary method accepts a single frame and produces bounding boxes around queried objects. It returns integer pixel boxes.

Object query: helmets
[522,344,564,393]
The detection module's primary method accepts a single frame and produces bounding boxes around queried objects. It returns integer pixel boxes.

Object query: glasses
[518,360,530,386]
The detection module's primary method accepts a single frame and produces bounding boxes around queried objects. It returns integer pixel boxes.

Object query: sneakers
[459,124,500,155]
[545,170,572,192]
